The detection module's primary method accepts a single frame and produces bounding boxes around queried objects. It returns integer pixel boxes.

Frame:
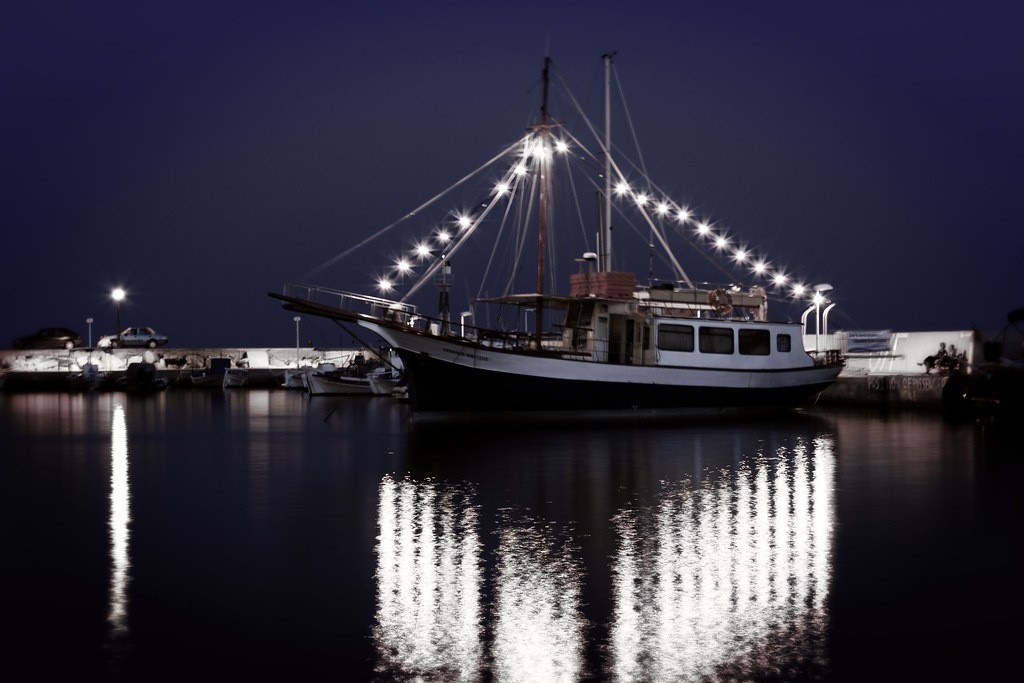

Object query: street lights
[110,287,125,348]
[292,315,301,368]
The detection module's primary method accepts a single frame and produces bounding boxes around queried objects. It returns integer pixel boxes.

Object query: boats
[271,53,847,414]
[281,352,412,401]
[77,354,252,392]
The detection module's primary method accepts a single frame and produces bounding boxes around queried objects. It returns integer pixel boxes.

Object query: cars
[97,326,170,351]
[11,327,83,351]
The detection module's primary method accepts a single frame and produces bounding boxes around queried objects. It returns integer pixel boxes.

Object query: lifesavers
[711,289,734,315]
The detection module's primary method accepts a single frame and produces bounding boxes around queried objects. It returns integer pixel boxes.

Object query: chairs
[938,352,962,374]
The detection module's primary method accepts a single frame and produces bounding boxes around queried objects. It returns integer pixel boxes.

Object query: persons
[160,355,166,368]
[916,343,956,375]
